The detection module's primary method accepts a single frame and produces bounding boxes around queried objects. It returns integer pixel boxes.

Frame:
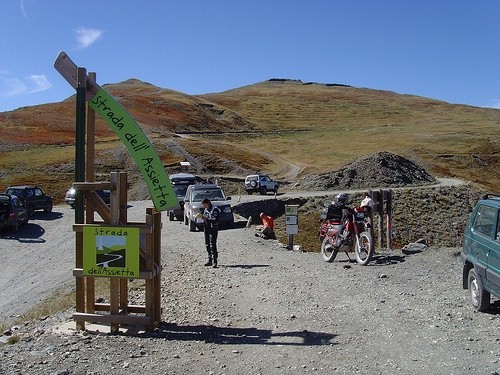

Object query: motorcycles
[320,203,374,267]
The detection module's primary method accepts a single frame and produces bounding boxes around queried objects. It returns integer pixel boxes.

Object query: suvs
[6,185,54,216]
[461,193,500,312]
[65,185,111,210]
[245,175,279,195]
[166,173,198,223]
[178,182,234,232]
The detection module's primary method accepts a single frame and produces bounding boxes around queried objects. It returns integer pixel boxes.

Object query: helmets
[359,194,374,211]
[336,193,348,206]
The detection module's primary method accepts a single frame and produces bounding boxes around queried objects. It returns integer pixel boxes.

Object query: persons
[201,198,221,268]
[255,212,276,239]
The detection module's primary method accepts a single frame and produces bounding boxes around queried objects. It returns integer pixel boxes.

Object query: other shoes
[213,263,218,268]
[204,261,212,266]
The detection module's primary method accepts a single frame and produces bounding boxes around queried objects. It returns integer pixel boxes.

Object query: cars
[0,193,29,235]
